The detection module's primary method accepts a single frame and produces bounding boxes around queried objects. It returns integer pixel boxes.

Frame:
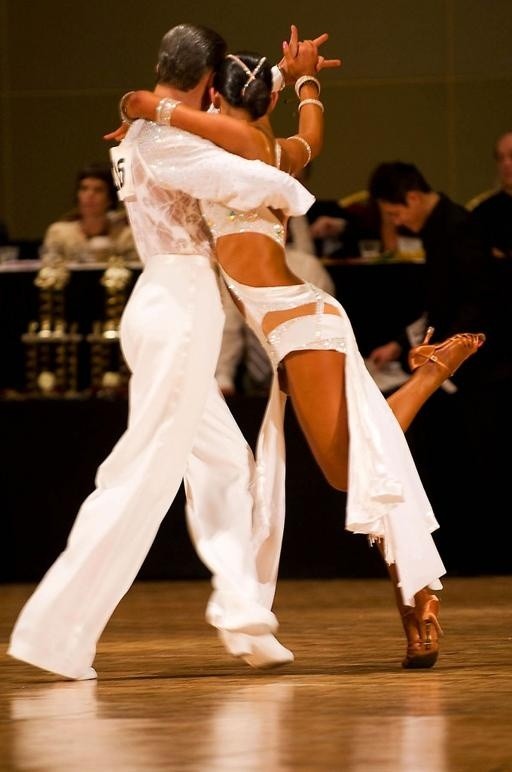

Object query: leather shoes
[70,666,98,683]
[239,630,295,671]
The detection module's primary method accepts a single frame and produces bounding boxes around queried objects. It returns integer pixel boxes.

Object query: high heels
[408,326,486,378]
[399,594,445,668]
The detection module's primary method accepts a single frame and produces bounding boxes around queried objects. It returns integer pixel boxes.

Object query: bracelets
[298,99,324,112]
[295,75,320,99]
[120,91,136,125]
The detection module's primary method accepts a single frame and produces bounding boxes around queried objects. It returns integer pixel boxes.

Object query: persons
[38,161,142,264]
[216,134,511,579]
[8,22,302,679]
[104,40,487,668]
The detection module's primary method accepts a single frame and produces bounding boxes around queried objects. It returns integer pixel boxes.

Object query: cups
[359,239,381,259]
